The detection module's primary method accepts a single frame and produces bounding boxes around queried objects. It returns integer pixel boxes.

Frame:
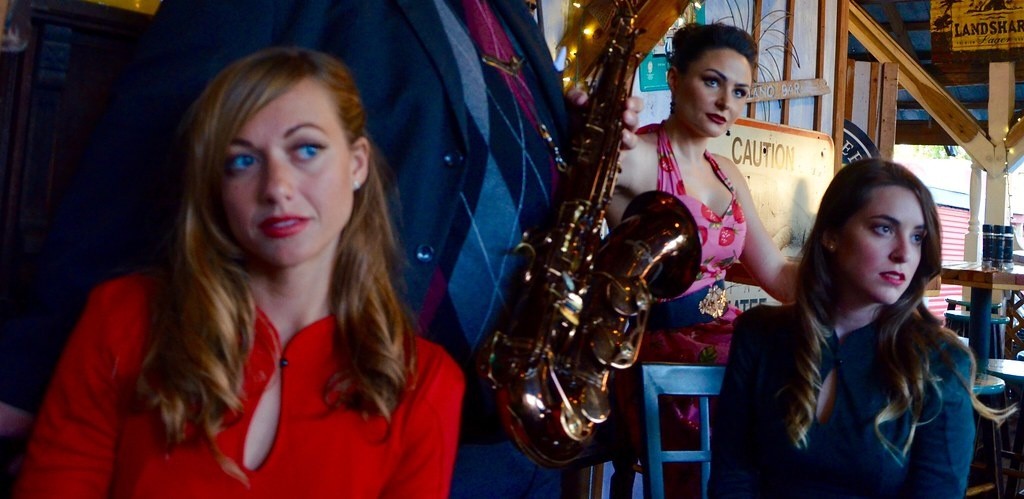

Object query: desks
[938,263,1024,375]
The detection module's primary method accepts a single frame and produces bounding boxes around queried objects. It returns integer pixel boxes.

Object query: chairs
[642,365,725,499]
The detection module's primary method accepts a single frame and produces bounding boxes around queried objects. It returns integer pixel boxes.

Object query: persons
[708,161,976,499]
[0,0,644,499]
[602,22,799,499]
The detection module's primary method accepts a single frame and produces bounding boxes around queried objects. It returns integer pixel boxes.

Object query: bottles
[993,224,1004,262]
[1004,225,1015,263]
[982,224,993,262]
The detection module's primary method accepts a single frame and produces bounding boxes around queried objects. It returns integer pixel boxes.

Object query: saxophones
[478,0,703,469]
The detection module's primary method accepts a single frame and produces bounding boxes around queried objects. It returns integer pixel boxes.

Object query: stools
[973,375,1006,499]
[944,310,1010,359]
[944,294,1003,336]
[987,359,1024,499]
